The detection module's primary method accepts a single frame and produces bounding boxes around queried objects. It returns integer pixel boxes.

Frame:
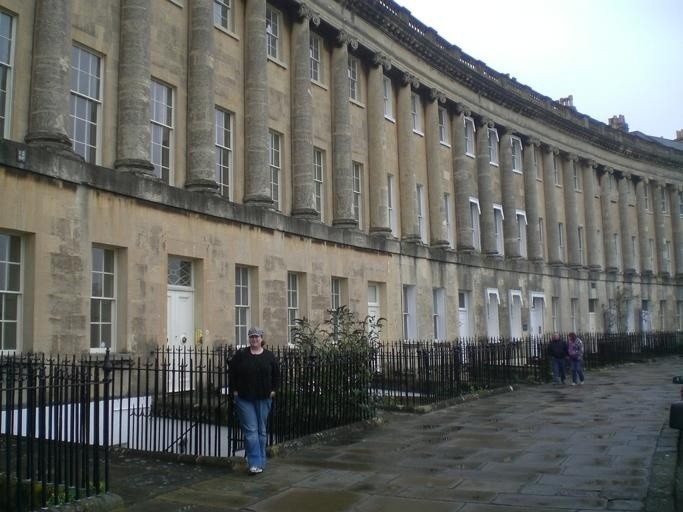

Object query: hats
[249,328,264,336]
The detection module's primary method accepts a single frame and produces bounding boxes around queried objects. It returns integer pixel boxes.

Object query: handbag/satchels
[571,354,583,360]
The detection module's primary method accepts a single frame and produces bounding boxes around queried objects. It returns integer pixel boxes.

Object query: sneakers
[249,466,263,473]
[572,380,584,385]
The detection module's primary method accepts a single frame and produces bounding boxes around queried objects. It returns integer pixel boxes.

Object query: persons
[548,333,570,385]
[229,327,280,474]
[568,332,585,385]
